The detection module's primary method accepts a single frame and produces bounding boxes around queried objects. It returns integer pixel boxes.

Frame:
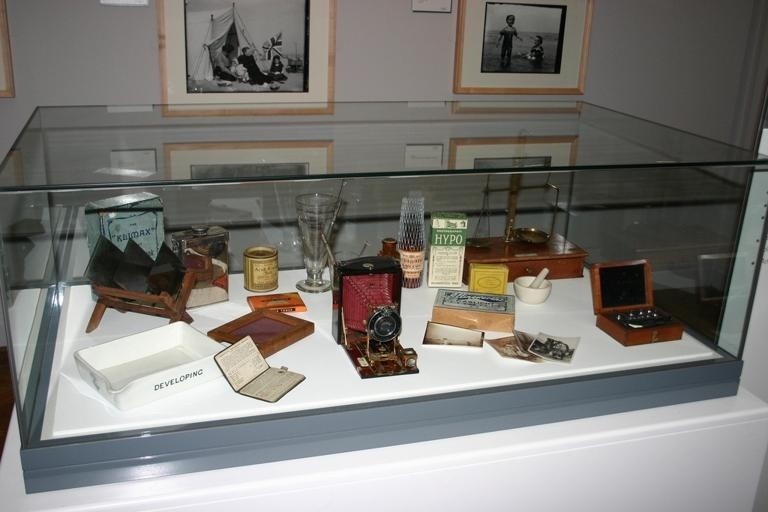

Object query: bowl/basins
[514,276,551,306]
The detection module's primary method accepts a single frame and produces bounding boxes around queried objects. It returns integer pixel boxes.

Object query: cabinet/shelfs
[0,99,768,512]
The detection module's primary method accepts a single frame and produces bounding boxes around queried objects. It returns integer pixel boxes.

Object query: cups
[242,246,277,293]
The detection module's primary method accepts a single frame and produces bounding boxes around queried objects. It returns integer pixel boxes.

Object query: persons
[495,14,522,71]
[526,36,545,69]
[215,44,237,84]
[267,54,290,81]
[237,48,273,85]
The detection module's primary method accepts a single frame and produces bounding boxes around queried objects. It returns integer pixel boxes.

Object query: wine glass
[296,189,337,294]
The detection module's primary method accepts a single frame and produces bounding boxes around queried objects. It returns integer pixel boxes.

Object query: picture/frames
[448,135,580,170]
[161,141,334,182]
[451,2,594,96]
[155,1,336,117]
[1,2,22,100]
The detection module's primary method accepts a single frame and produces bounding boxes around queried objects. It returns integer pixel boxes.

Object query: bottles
[171,224,227,311]
[397,211,427,287]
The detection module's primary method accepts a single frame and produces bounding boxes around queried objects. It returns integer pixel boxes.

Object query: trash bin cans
[1,237,35,287]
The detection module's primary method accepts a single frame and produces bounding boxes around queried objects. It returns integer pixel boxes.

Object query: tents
[192,6,271,82]
